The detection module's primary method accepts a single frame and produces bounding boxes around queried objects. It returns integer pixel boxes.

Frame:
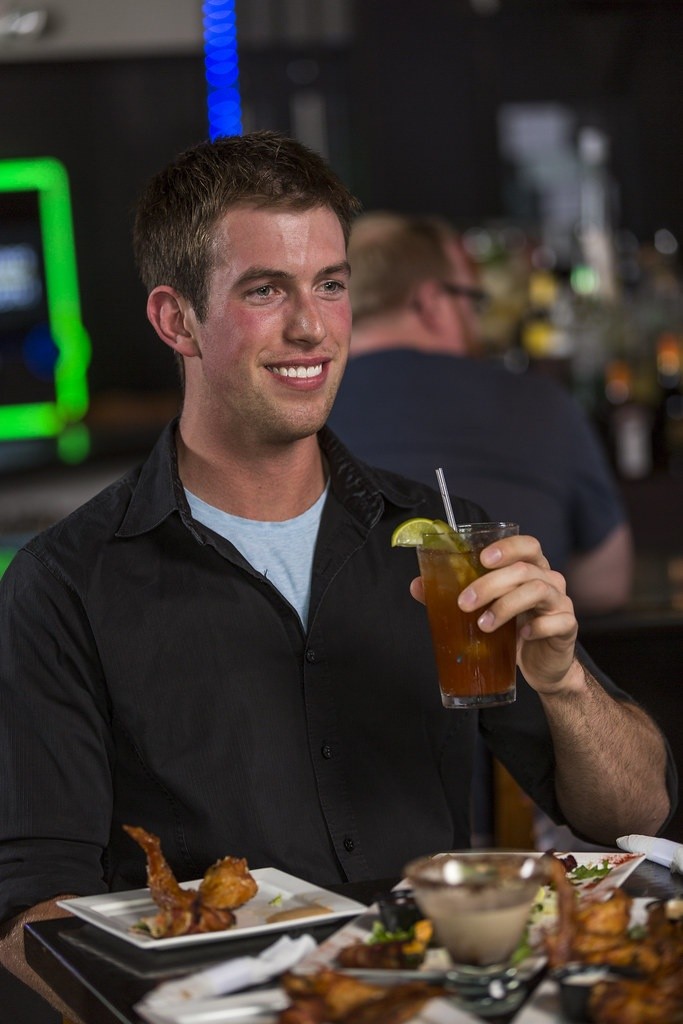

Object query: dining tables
[24,857,683,1024]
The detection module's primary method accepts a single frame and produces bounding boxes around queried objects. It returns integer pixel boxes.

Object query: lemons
[390,518,438,548]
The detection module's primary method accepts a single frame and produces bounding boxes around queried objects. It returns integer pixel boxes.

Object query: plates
[291,850,645,993]
[56,865,370,951]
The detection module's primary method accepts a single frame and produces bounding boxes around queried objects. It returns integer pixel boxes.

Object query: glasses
[441,284,488,305]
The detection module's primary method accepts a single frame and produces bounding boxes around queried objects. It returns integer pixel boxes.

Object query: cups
[403,847,544,966]
[415,521,519,710]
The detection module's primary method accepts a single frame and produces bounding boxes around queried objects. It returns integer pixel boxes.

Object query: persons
[0,135,674,1024]
[322,206,628,845]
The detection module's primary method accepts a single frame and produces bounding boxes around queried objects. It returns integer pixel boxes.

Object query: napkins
[130,934,317,1024]
